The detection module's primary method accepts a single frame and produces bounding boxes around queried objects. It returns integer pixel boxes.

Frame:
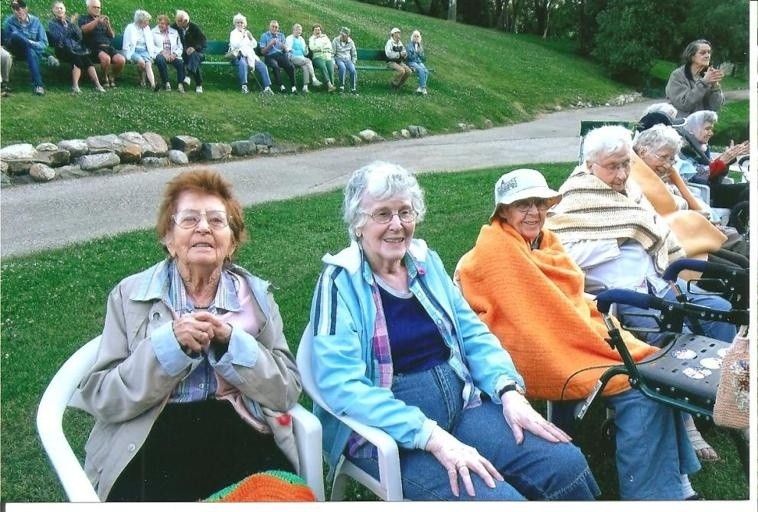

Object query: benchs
[12,35,434,76]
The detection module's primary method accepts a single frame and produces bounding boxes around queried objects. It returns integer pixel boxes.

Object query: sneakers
[184,76,192,86]
[164,81,172,92]
[176,84,185,94]
[280,84,287,94]
[351,89,360,96]
[301,87,312,95]
[313,80,324,86]
[290,87,299,95]
[417,87,429,95]
[101,77,110,88]
[153,80,162,93]
[240,85,249,94]
[328,85,338,94]
[34,85,46,96]
[339,87,345,95]
[71,85,83,96]
[139,79,147,89]
[263,86,275,96]
[110,78,117,88]
[96,85,106,94]
[196,86,204,95]
[0,83,14,98]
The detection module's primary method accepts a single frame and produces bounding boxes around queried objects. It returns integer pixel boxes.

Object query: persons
[229,13,358,95]
[385,27,413,91]
[309,160,603,501]
[542,40,748,461]
[78,168,318,502]
[405,29,430,95]
[1,0,206,97]
[452,168,704,501]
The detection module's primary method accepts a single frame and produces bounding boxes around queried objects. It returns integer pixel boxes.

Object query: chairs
[547,275,616,437]
[297,321,412,502]
[37,333,326,502]
[687,178,734,226]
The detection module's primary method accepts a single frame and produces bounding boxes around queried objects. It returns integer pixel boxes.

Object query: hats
[340,26,351,37]
[390,27,401,34]
[487,168,563,225]
[9,0,28,10]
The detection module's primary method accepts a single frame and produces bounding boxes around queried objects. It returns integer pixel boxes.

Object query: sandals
[677,413,723,464]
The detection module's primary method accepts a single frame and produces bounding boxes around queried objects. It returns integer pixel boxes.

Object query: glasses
[646,145,678,166]
[592,158,637,172]
[359,205,420,225]
[501,196,556,211]
[169,208,234,230]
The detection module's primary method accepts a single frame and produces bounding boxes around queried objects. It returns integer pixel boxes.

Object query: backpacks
[61,33,92,57]
